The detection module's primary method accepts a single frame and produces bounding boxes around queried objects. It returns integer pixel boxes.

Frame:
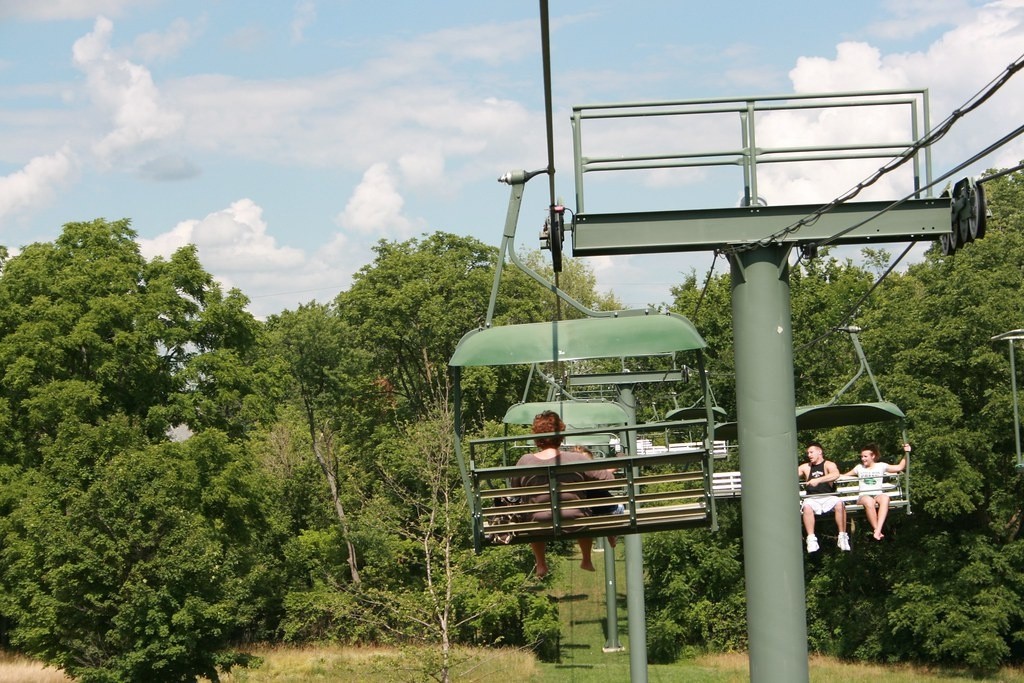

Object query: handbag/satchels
[486,497,522,545]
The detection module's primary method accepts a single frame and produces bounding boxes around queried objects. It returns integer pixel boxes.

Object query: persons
[511,410,624,576]
[841,444,911,539]
[798,443,851,553]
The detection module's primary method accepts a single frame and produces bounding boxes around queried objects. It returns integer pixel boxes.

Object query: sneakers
[838,533,851,550]
[806,537,819,553]
